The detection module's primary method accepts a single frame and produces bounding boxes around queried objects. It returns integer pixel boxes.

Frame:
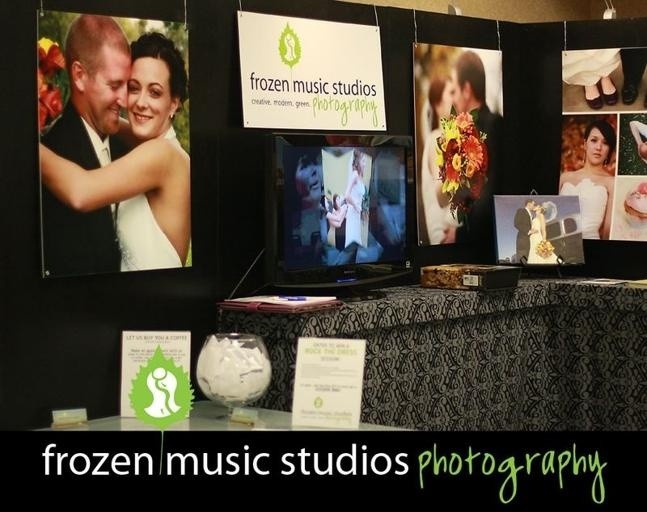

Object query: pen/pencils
[267,295,308,301]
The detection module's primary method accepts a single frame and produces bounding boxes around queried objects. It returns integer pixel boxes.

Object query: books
[215,294,345,315]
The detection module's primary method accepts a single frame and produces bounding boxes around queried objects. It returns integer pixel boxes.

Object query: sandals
[584,76,618,109]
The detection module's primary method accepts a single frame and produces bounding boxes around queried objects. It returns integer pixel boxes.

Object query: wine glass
[198,331,275,424]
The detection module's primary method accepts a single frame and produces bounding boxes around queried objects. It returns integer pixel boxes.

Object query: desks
[45,401,415,434]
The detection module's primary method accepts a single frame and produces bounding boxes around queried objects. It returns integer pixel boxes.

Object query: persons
[523,204,562,264]
[419,75,461,245]
[619,49,646,108]
[37,31,193,272]
[273,142,368,267]
[512,198,537,262]
[554,118,615,241]
[628,119,646,163]
[562,49,618,109]
[426,50,505,246]
[41,14,132,278]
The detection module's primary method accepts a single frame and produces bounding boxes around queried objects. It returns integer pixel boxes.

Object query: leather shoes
[621,80,638,105]
